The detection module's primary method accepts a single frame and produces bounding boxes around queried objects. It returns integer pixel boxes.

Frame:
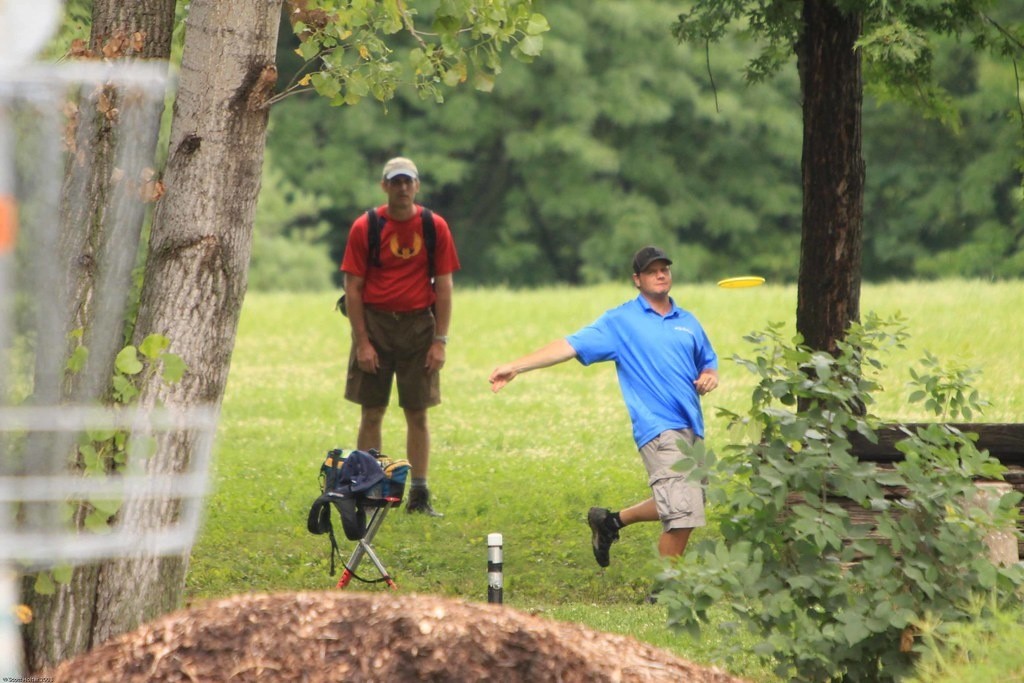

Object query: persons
[343,155,461,519]
[488,246,719,606]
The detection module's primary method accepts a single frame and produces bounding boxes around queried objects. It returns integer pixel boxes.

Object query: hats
[384,156,419,180]
[632,247,673,272]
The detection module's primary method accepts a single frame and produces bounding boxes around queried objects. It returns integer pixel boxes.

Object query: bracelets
[432,335,448,345]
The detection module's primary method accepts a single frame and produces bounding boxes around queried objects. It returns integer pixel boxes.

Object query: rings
[704,382,707,386]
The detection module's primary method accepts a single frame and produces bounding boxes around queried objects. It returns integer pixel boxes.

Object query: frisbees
[717,277,765,288]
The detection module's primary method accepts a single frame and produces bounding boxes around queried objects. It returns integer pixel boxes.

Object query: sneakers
[588,507,619,567]
[407,485,431,515]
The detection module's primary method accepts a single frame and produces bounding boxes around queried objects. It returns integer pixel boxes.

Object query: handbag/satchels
[317,448,411,509]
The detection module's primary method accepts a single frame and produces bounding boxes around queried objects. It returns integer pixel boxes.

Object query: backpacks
[307,450,384,540]
